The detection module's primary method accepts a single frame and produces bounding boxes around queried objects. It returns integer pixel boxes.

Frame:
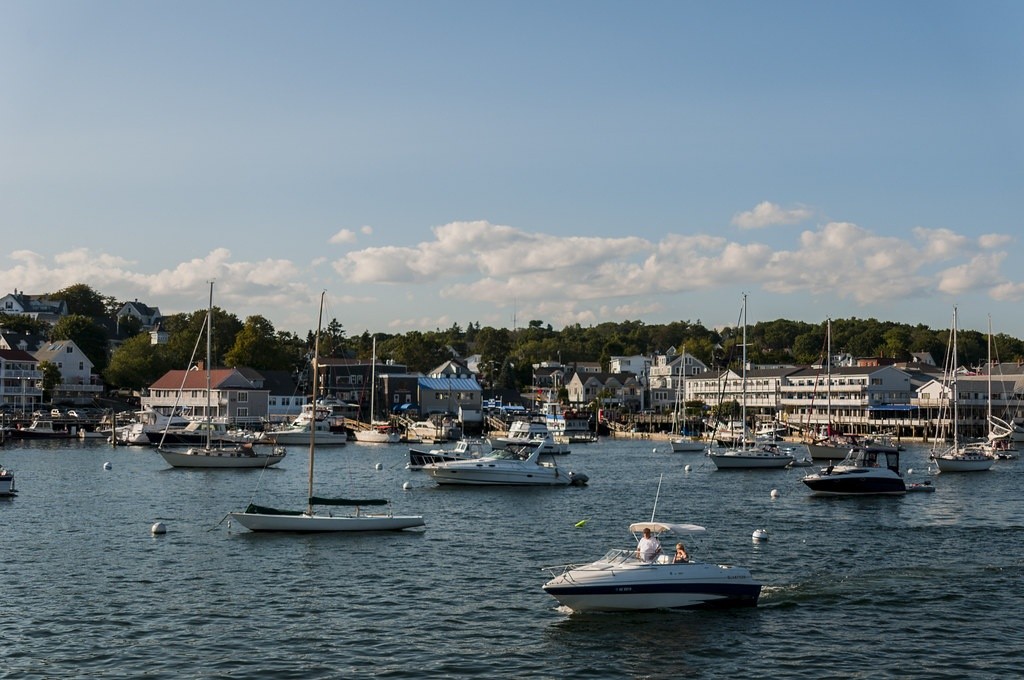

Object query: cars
[64,408,87,418]
[83,408,101,418]
[33,410,49,419]
[0,409,23,419]
[51,409,64,418]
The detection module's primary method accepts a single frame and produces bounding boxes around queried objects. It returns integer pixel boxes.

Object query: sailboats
[708,294,793,469]
[156,281,287,467]
[800,318,897,459]
[352,337,400,443]
[967,313,1019,458]
[930,307,995,471]
[226,290,426,532]
[670,344,705,451]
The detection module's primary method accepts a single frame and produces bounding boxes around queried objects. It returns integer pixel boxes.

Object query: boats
[421,432,572,485]
[541,472,768,610]
[404,439,493,470]
[803,447,907,494]
[491,374,598,442]
[481,421,571,454]
[301,394,360,418]
[264,404,347,444]
[758,424,787,435]
[99,410,190,444]
[11,421,69,437]
[715,420,752,446]
[399,418,462,443]
[0,463,19,497]
[145,416,257,446]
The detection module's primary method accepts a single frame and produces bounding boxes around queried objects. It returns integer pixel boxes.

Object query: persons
[636,528,661,563]
[674,543,689,563]
